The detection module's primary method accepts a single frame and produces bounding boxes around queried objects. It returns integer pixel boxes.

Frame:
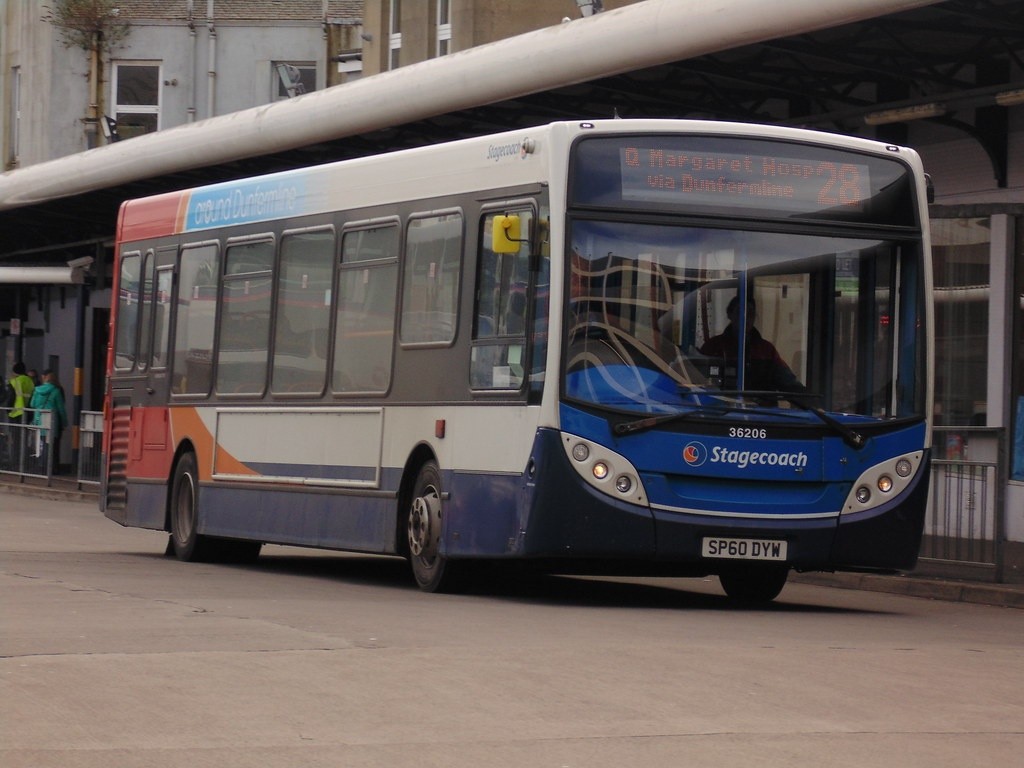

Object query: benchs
[117,303,292,358]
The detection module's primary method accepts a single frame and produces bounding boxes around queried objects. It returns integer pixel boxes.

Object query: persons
[29,370,68,475]
[701,296,819,410]
[7,363,41,470]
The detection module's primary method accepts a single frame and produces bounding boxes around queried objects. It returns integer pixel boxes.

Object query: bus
[103,119,936,605]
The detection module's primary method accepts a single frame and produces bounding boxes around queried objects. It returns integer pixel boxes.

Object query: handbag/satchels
[26,422,36,447]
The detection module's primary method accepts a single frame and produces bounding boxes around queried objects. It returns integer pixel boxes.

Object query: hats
[13,362,26,374]
